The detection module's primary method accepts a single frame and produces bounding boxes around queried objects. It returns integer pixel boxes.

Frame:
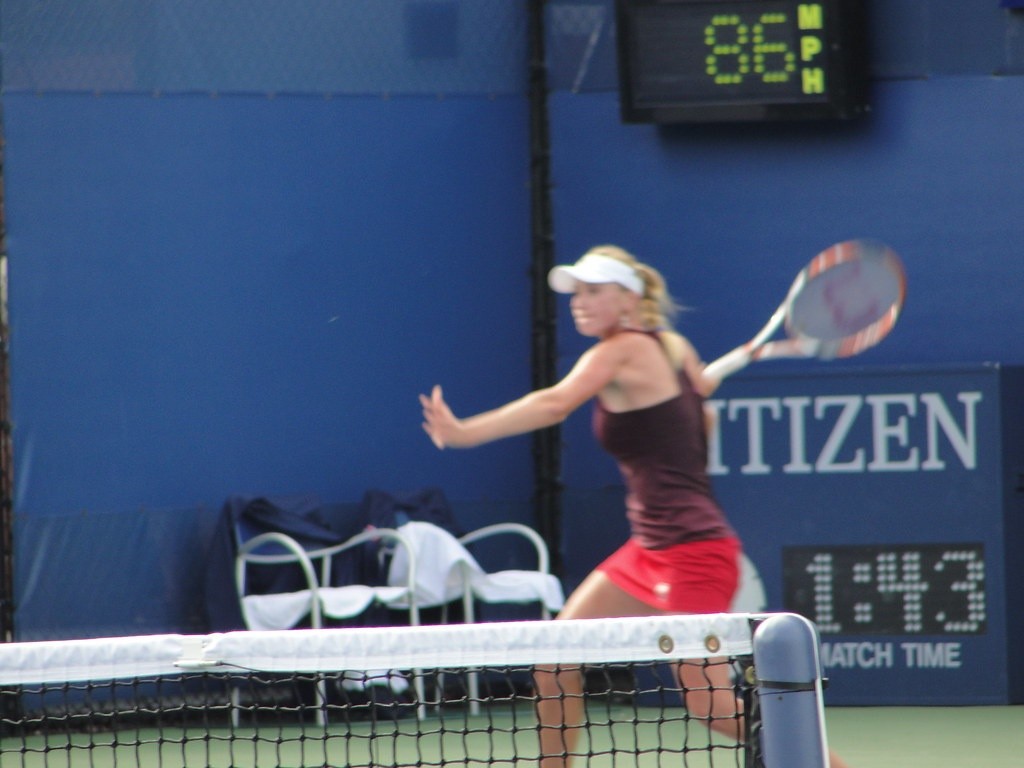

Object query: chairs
[202,487,567,727]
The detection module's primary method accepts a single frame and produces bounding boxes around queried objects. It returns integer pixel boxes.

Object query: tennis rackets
[704,241,906,386]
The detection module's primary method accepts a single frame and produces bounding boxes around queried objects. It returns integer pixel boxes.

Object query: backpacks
[340,487,473,586]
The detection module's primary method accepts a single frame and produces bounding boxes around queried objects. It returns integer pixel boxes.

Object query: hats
[547,254,645,297]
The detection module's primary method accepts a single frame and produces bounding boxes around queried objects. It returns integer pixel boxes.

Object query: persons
[419,246,845,768]
